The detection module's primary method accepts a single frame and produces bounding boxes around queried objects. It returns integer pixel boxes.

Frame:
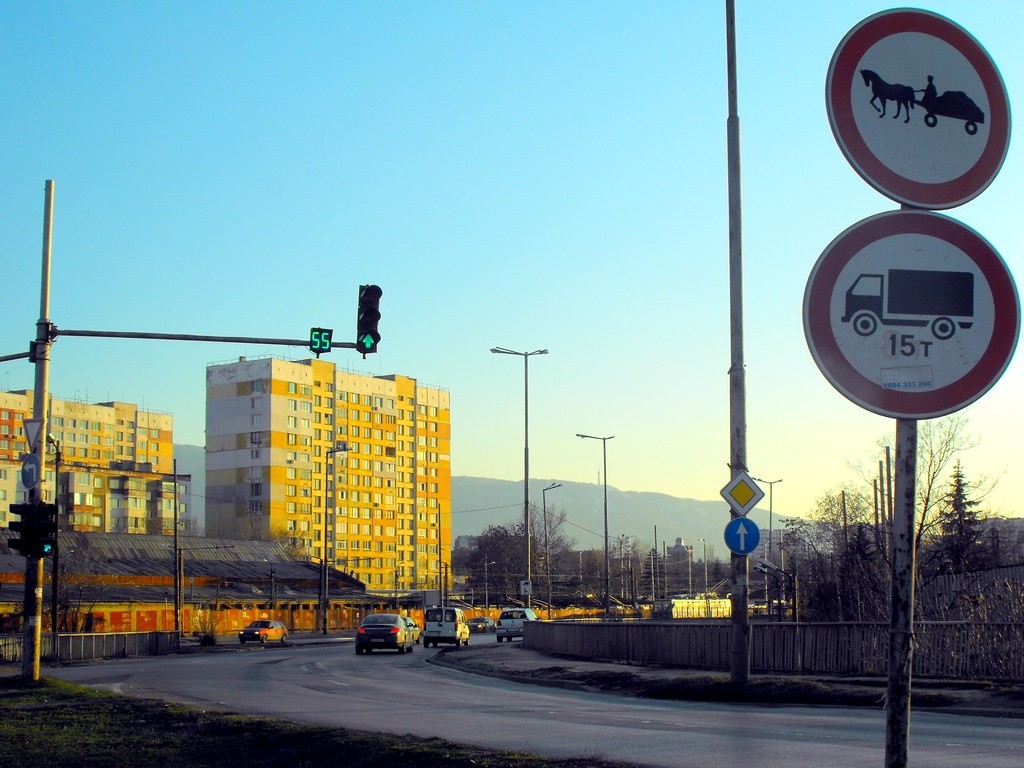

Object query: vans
[423,607,469,647]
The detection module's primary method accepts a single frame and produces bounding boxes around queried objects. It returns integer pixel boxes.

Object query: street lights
[490,346,549,607]
[542,483,563,620]
[752,477,782,562]
[687,549,693,598]
[485,562,495,609]
[620,535,635,599]
[753,565,783,621]
[437,560,448,607]
[759,558,796,622]
[576,433,614,614]
[323,447,352,634]
[263,558,275,596]
[394,562,405,609]
[698,538,708,593]
[46,435,60,635]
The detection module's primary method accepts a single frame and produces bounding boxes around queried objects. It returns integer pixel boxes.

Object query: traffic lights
[8,504,25,551]
[309,327,333,353]
[360,285,383,352]
[37,504,56,554]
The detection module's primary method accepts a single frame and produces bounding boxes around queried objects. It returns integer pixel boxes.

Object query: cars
[496,608,541,642]
[355,614,414,655]
[239,620,288,643]
[403,617,421,644]
[467,617,496,632]
